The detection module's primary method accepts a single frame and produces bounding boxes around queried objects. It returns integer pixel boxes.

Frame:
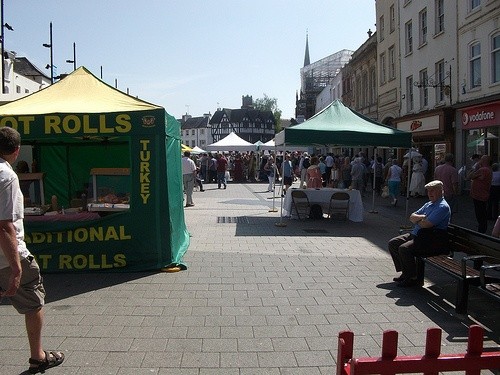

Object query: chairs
[289,190,314,222]
[327,193,350,225]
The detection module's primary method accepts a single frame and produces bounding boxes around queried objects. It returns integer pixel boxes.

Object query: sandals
[28,349,64,371]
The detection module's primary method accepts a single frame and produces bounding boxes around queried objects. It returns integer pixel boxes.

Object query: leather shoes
[393,277,424,287]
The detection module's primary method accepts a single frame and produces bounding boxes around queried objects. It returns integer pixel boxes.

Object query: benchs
[399,223,500,318]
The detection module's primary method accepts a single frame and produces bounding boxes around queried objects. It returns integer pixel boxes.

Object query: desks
[282,186,364,222]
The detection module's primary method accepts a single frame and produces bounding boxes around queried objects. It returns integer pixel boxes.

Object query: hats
[424,179,443,188]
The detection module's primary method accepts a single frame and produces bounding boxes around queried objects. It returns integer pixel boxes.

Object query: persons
[16,160,31,203]
[467,156,493,234]
[0,126,64,373]
[388,180,451,287]
[181,152,197,207]
[182,149,500,208]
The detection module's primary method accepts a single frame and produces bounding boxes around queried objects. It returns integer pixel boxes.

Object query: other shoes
[392,198,397,205]
[268,187,274,192]
[223,185,226,189]
[186,201,195,206]
[246,178,263,183]
[283,188,286,195]
[217,186,221,189]
[200,188,205,192]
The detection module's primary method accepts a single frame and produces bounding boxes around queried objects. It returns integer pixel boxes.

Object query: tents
[181,132,313,184]
[190,146,207,154]
[181,144,192,152]
[0,66,191,274]
[269,98,413,229]
